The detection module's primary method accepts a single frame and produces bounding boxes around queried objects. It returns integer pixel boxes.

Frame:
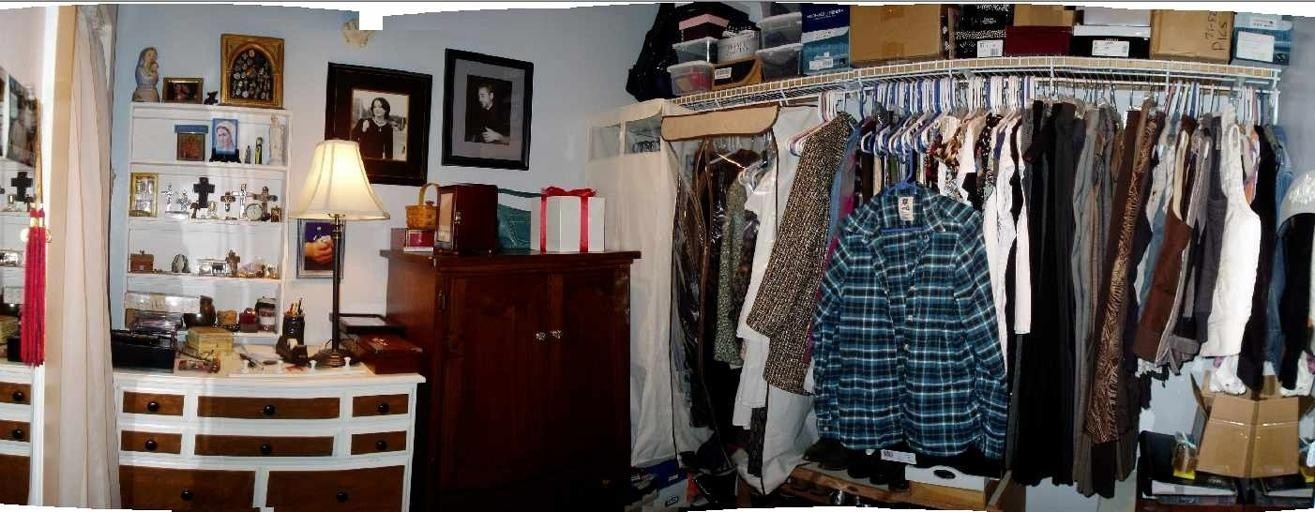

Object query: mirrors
[0,66,39,503]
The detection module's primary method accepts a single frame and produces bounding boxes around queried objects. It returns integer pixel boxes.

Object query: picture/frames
[210,117,239,157]
[219,33,284,109]
[160,76,204,103]
[295,216,348,279]
[128,171,160,217]
[439,48,534,172]
[322,61,435,187]
[326,312,406,334]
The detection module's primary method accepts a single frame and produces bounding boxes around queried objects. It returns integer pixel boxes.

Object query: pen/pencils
[240,353,264,369]
[286,298,303,315]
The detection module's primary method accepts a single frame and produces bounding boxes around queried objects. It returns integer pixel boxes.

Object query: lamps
[289,137,392,369]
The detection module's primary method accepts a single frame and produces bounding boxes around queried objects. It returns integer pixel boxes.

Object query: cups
[277,300,304,362]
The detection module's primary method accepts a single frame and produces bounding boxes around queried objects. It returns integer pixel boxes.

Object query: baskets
[403,182,443,231]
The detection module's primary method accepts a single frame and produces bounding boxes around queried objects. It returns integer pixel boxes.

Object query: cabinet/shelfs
[119,102,292,345]
[1,361,38,503]
[729,445,1026,511]
[107,344,427,512]
[1129,455,1313,512]
[377,247,642,511]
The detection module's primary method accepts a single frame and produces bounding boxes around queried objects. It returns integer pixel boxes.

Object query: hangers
[705,129,777,193]
[859,75,1118,165]
[1127,78,1273,162]
[785,89,854,158]
[879,143,924,233]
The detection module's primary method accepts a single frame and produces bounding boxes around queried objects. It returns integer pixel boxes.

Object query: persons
[135,47,159,88]
[350,96,392,162]
[167,83,195,100]
[10,96,28,162]
[305,222,333,270]
[215,121,237,152]
[465,82,509,145]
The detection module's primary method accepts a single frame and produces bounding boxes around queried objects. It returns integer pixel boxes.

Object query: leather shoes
[800,435,908,495]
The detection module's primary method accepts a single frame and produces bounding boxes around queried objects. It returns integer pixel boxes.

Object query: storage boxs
[667,1,851,95]
[849,4,943,68]
[1251,372,1300,479]
[941,0,1295,86]
[1185,367,1256,480]
[530,184,605,253]
